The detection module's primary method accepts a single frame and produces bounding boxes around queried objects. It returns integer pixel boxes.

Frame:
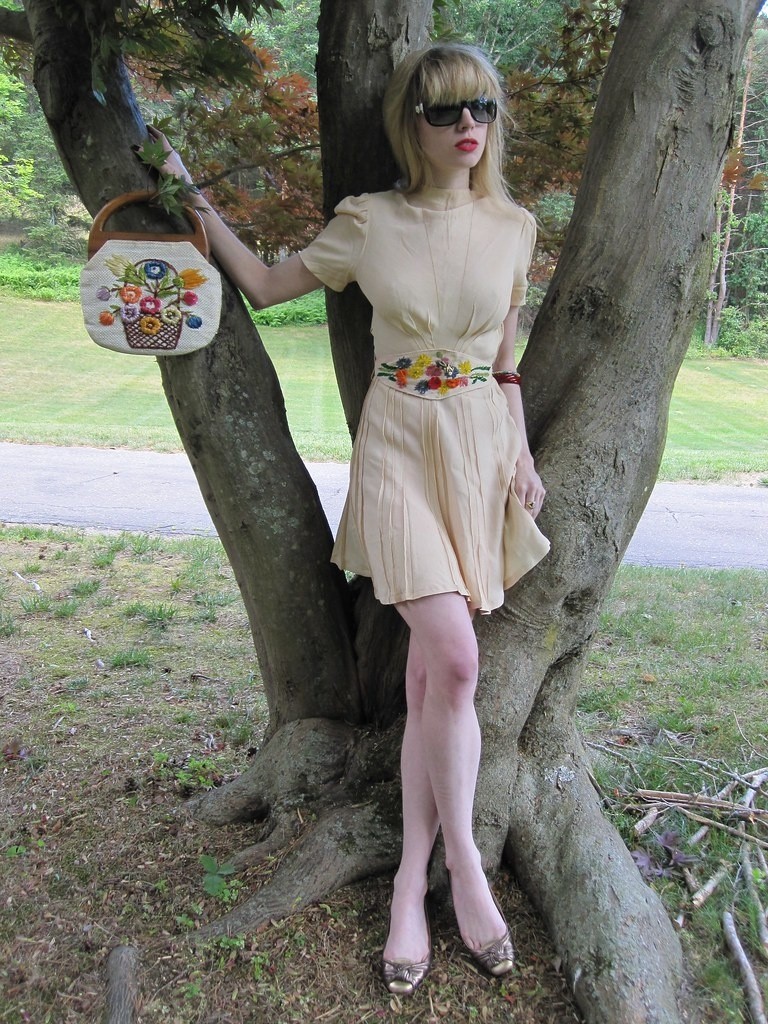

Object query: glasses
[415,96,497,127]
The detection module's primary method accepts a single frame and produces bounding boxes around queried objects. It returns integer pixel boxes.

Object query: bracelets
[492,371,521,384]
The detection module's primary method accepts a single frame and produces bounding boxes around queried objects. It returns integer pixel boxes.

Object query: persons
[131,44,552,993]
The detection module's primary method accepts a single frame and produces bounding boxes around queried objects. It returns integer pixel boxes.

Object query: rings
[525,501,535,509]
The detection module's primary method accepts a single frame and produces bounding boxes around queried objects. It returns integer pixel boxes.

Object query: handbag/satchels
[80,191,222,356]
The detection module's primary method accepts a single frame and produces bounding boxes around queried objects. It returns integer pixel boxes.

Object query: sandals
[382,892,431,993]
[447,867,515,976]
